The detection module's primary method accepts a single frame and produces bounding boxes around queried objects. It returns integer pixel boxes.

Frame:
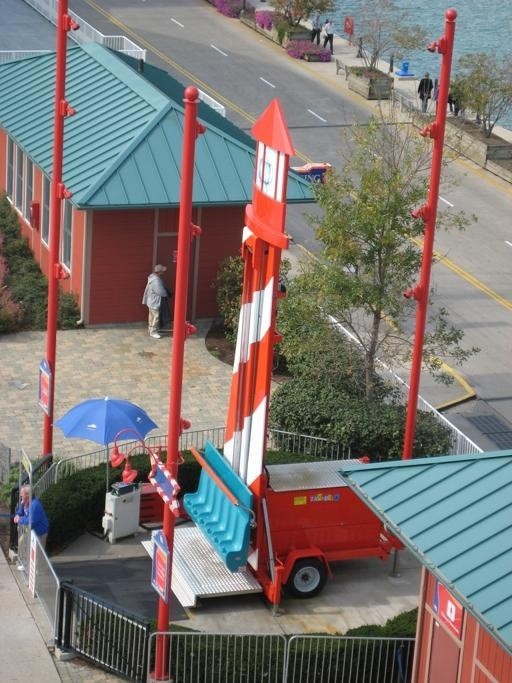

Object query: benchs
[391,90,420,119]
[336,59,350,80]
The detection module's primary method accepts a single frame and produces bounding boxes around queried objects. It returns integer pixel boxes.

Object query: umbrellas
[51,395,160,494]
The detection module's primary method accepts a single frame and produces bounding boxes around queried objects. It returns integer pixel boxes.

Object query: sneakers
[149,332,162,339]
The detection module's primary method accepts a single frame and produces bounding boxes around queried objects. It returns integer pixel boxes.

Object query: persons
[13,482,49,575]
[447,79,456,114]
[321,17,336,54]
[452,78,465,118]
[311,13,323,45]
[142,263,170,338]
[417,71,433,111]
[432,78,441,115]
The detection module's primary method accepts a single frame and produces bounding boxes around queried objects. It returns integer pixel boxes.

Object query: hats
[153,264,167,273]
